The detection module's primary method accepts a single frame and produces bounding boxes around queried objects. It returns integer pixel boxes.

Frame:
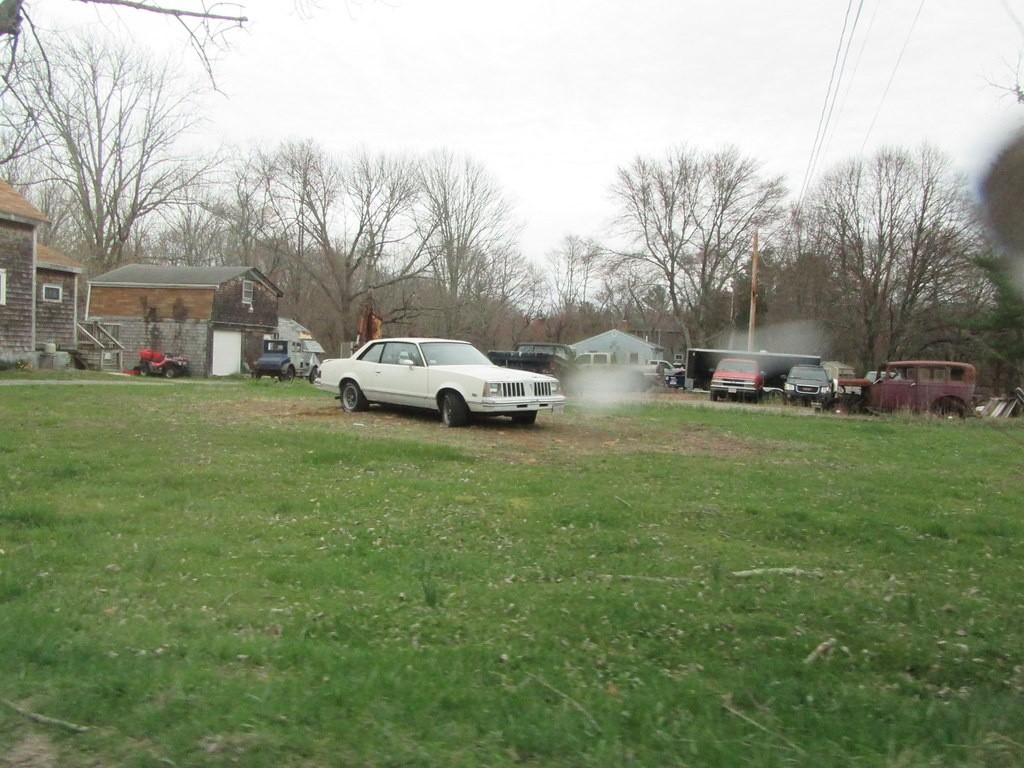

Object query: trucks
[572,351,664,384]
[489,340,579,396]
[646,360,676,379]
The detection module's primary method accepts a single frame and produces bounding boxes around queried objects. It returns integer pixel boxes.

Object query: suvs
[707,357,767,405]
[779,362,836,411]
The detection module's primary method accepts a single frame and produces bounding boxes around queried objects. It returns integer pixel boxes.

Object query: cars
[310,337,566,428]
[132,352,188,379]
[249,337,319,384]
[864,372,902,383]
[835,361,978,424]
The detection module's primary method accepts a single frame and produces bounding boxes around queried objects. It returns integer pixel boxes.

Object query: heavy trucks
[685,346,856,395]
